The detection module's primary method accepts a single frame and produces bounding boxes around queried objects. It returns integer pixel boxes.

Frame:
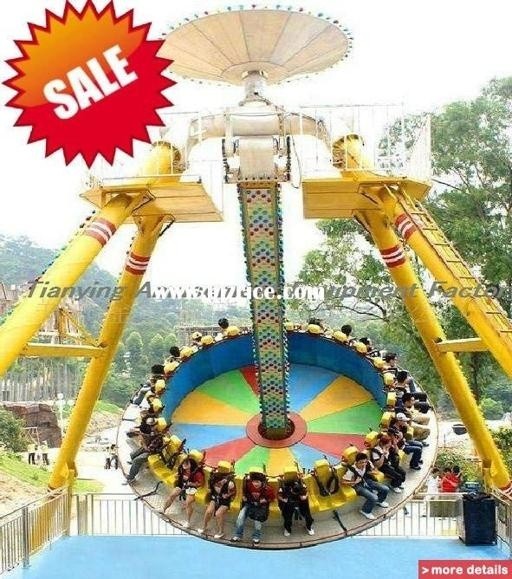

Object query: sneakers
[229,536,240,544]
[284,528,290,537]
[390,484,405,493]
[306,527,315,536]
[359,510,374,519]
[376,502,389,508]
[252,539,260,547]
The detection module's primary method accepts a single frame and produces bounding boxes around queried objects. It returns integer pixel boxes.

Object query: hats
[397,413,410,422]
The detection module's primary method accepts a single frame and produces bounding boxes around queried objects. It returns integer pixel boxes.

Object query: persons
[27,437,38,467]
[38,439,51,467]
[105,312,466,547]
